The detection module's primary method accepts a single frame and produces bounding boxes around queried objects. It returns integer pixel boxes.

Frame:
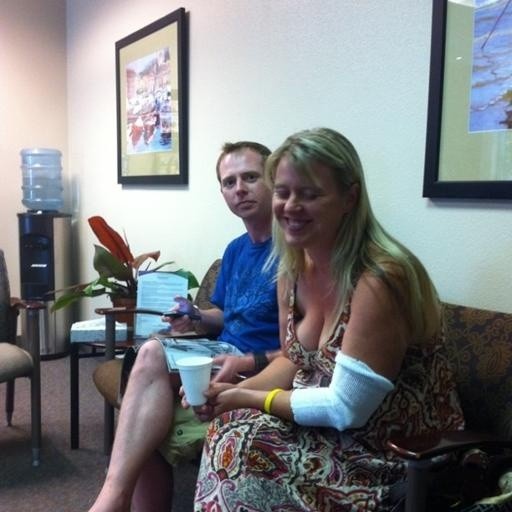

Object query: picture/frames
[114,5,190,187]
[420,0,511,201]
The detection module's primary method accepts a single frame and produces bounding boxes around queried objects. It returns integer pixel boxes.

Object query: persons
[193,129,458,511]
[86,141,287,511]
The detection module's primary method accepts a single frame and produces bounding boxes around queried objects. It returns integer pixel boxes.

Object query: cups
[174,356,215,408]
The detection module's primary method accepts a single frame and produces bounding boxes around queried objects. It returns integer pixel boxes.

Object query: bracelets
[264,388,283,415]
[252,351,268,371]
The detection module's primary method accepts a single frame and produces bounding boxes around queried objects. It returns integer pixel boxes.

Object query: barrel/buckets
[20,148,64,209]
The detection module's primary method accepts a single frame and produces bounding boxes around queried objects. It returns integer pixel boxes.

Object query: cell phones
[163,312,203,321]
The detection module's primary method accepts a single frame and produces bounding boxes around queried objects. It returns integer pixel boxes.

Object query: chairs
[383,295,511,509]
[0,249,48,464]
[85,256,220,456]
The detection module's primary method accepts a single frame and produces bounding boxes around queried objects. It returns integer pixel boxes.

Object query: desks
[63,314,125,451]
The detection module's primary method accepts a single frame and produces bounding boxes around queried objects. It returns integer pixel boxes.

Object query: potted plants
[40,210,199,325]
